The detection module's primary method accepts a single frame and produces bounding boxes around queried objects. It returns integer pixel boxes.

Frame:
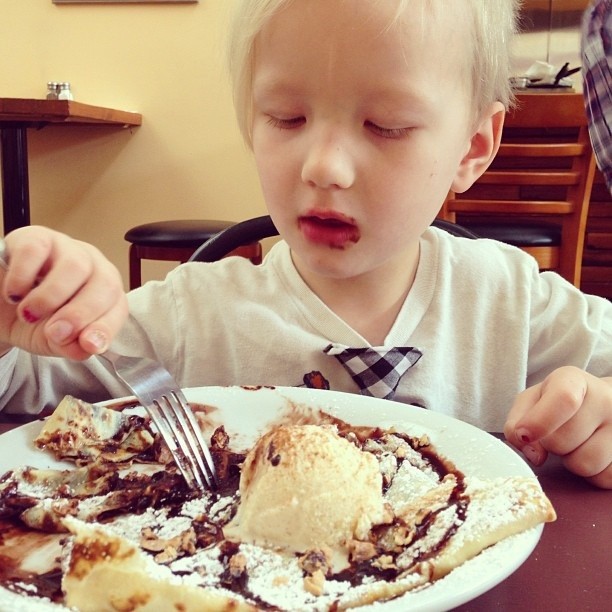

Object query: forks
[0,234,217,497]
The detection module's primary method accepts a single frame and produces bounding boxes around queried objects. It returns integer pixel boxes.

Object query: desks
[1,97,142,242]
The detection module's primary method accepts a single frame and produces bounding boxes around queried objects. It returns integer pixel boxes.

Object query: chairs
[444,93,597,290]
[187,215,480,262]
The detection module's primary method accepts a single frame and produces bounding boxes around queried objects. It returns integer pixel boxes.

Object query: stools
[124,220,262,290]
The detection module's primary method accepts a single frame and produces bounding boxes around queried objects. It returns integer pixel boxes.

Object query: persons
[0,0,612,491]
